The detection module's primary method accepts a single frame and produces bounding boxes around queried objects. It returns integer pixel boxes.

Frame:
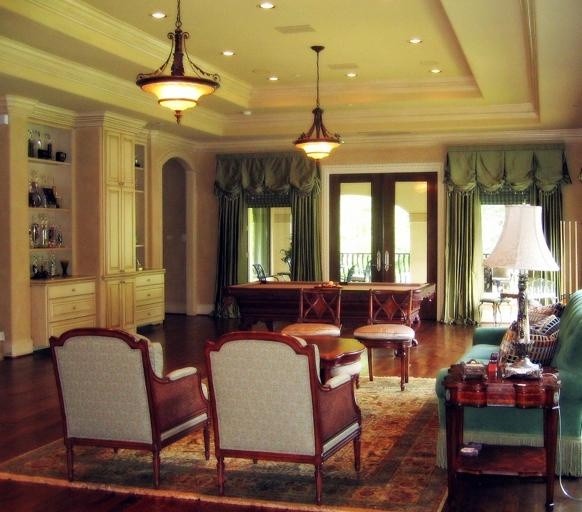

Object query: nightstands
[0,94,98,359]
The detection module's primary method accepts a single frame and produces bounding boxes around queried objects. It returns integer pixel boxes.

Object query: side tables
[441,360,562,511]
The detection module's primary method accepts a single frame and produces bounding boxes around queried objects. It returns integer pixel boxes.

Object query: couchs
[434,288,581,476]
[49,327,211,488]
[204,332,362,506]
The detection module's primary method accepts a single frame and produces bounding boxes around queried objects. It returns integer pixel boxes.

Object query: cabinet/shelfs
[100,109,134,332]
[133,123,166,328]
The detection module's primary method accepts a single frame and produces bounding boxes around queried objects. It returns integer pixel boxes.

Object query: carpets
[0,373,449,512]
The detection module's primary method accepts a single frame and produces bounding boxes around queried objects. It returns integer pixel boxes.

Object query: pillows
[499,303,564,367]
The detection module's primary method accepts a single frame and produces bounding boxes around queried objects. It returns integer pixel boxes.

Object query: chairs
[281,287,341,338]
[349,261,371,283]
[253,264,279,283]
[353,288,415,391]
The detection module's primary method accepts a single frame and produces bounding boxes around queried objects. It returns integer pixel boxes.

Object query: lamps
[481,200,561,379]
[135,0,221,121]
[293,45,342,162]
